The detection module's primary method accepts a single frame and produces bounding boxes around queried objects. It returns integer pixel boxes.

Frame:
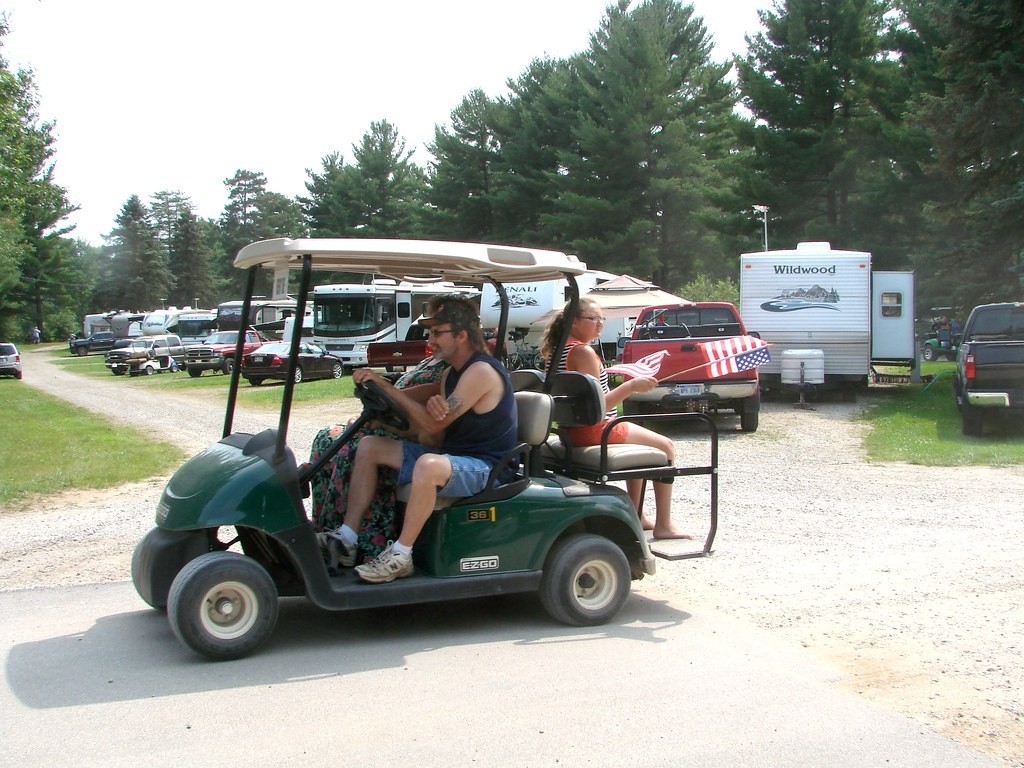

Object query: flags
[606,347,671,380]
[698,334,770,378]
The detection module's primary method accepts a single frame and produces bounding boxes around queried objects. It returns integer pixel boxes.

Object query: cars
[238,341,344,388]
[0,342,22,380]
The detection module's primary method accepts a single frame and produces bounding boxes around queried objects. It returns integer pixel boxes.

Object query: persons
[541,296,695,540]
[932,318,961,335]
[312,292,520,583]
[33,326,41,344]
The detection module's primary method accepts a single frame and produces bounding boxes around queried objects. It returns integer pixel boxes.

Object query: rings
[368,372,371,375]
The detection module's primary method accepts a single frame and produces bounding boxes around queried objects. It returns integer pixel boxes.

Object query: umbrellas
[529,274,692,327]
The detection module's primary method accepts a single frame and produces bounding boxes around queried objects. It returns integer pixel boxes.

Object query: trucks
[738,241,917,403]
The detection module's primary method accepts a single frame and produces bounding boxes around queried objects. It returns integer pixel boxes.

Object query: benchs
[509,369,668,471]
[396,390,555,512]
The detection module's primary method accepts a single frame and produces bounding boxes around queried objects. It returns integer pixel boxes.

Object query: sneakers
[314,525,359,565]
[354,539,414,583]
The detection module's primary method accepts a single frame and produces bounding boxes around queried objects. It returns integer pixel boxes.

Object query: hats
[418,306,481,329]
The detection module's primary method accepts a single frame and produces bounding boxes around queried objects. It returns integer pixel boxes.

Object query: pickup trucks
[70,331,138,356]
[616,299,767,433]
[955,301,1024,438]
[105,334,203,376]
[185,329,277,378]
[367,312,508,372]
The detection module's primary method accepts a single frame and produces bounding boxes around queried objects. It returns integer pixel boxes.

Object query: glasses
[428,329,456,338]
[579,315,607,324]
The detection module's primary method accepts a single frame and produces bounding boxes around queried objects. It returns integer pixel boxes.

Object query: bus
[176,311,242,346]
[308,279,483,369]
[217,295,323,333]
